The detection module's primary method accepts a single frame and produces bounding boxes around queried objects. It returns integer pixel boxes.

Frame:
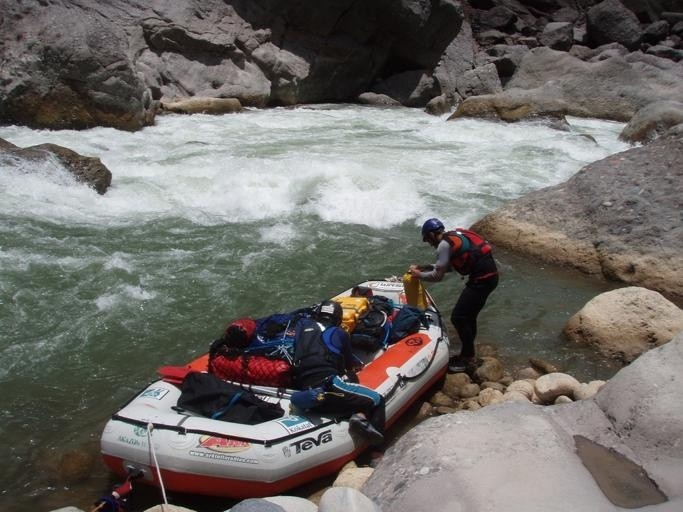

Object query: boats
[98,275,452,499]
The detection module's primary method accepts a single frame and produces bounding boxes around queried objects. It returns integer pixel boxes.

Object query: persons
[294,300,387,443]
[410,217,499,373]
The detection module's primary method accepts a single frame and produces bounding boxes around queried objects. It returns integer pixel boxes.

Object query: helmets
[314,299,343,326]
[422,218,445,243]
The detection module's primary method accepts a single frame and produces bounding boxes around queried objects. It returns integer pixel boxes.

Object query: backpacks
[348,294,421,353]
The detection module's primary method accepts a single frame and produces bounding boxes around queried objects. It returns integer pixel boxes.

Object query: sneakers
[446,354,476,373]
[348,413,384,451]
